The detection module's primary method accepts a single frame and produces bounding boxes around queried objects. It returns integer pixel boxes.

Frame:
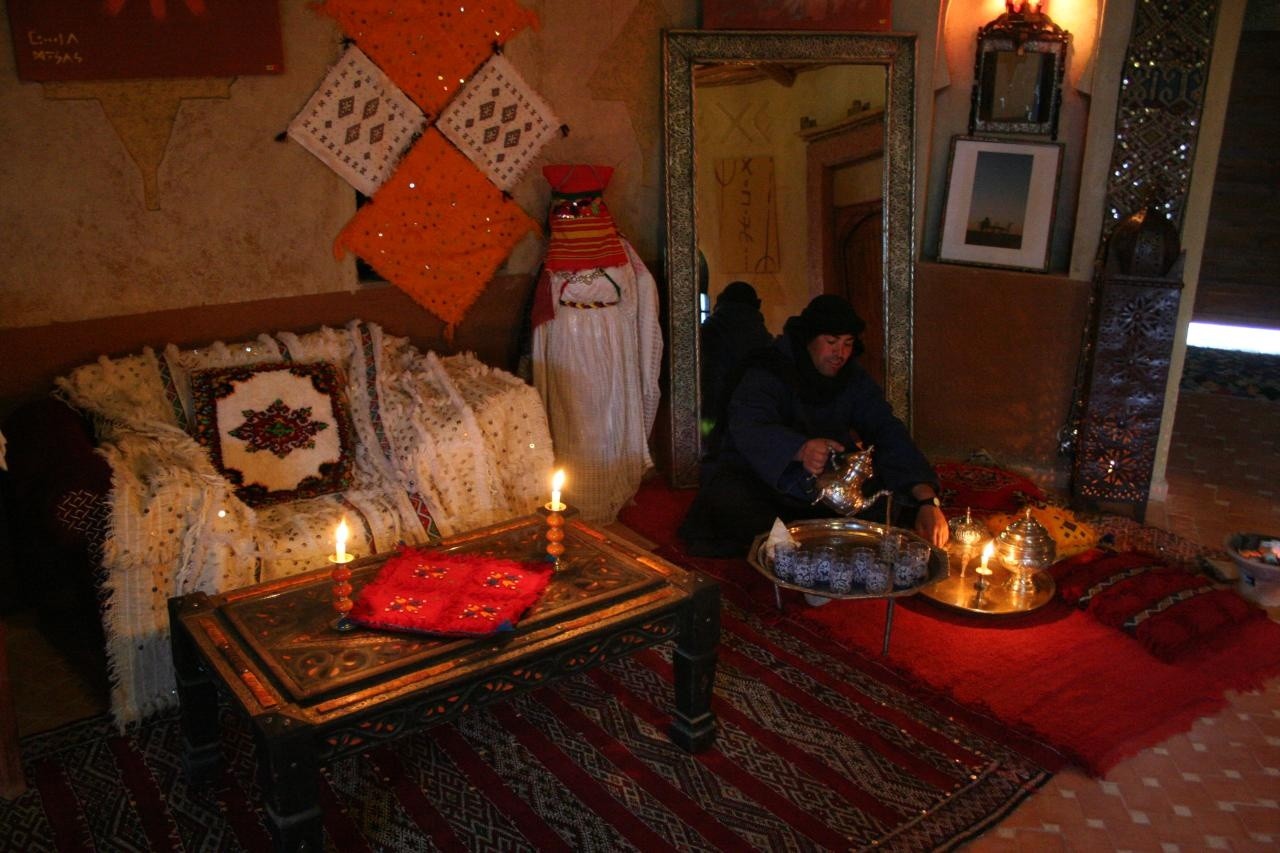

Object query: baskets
[1224,532,1280,608]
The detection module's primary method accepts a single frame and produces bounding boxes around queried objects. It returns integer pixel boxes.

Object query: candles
[335,516,349,560]
[981,538,996,569]
[550,470,566,510]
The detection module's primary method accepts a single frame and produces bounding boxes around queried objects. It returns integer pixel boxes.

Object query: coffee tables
[169,508,718,853]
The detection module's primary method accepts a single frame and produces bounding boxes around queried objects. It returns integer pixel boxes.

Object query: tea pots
[806,444,894,517]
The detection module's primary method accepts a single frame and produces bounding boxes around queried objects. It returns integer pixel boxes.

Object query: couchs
[37,324,557,725]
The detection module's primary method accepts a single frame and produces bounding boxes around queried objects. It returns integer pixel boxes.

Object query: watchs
[918,496,941,508]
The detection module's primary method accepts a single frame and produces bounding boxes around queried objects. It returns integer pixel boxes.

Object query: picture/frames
[938,135,1065,274]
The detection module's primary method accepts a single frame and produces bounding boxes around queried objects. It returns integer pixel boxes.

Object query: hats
[787,293,865,333]
[717,281,761,309]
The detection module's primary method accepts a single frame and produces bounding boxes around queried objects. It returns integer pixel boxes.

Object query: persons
[714,294,950,550]
[699,282,774,431]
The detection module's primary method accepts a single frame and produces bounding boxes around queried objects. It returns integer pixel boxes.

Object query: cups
[829,558,854,596]
[881,528,931,592]
[849,546,876,586]
[792,549,820,587]
[862,558,891,594]
[812,543,838,585]
[773,540,797,583]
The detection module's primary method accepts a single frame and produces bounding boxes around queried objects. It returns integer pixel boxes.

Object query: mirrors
[663,26,915,482]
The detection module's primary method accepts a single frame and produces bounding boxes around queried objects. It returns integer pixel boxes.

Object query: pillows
[193,363,355,504]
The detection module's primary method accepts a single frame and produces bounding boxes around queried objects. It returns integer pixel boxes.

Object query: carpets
[0,556,1067,853]
[635,461,1280,778]
